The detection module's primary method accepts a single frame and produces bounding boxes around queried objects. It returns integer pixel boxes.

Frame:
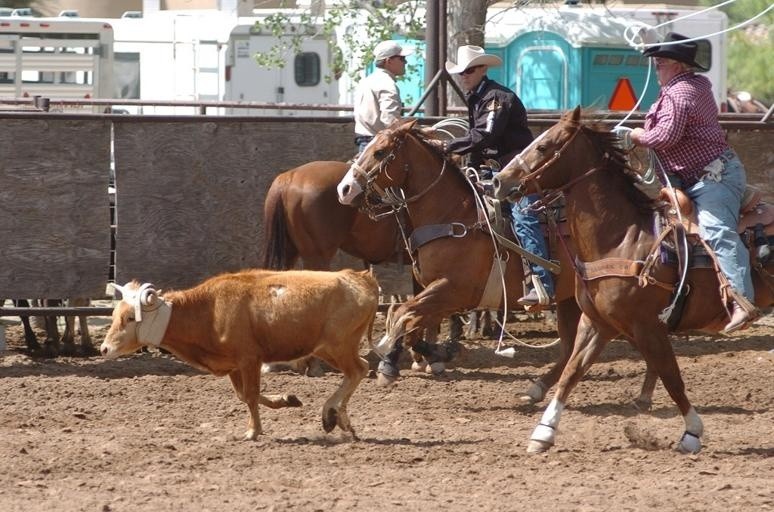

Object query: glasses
[458,65,485,75]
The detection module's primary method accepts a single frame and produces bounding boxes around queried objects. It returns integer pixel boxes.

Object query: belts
[680,151,736,189]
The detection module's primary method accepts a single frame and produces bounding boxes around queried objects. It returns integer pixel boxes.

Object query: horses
[337,117,659,412]
[259,160,503,375]
[491,106,774,455]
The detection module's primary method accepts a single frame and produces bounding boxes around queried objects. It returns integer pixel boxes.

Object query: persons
[350,40,435,155]
[607,32,760,335]
[420,44,556,305]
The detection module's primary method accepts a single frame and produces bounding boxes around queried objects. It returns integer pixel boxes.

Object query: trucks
[90,10,344,117]
[0,11,117,115]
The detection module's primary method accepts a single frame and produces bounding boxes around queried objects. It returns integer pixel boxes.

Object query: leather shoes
[724,299,763,334]
[516,284,556,306]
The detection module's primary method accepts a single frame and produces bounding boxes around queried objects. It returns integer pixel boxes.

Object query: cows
[100,269,419,441]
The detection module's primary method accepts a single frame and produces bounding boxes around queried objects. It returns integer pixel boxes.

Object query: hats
[641,33,704,69]
[372,38,418,61]
[444,44,504,74]
